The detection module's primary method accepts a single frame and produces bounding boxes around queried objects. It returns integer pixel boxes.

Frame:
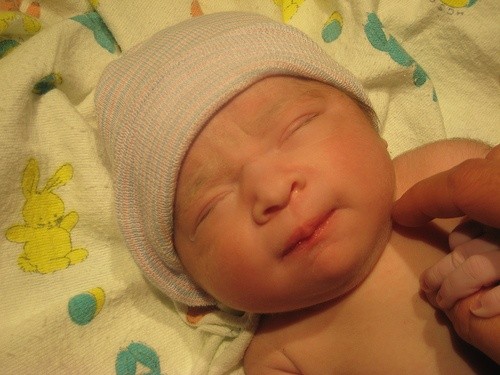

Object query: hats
[97,11,385,307]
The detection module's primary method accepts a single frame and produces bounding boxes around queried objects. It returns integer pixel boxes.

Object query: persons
[90,11,500,375]
[389,143,499,361]
